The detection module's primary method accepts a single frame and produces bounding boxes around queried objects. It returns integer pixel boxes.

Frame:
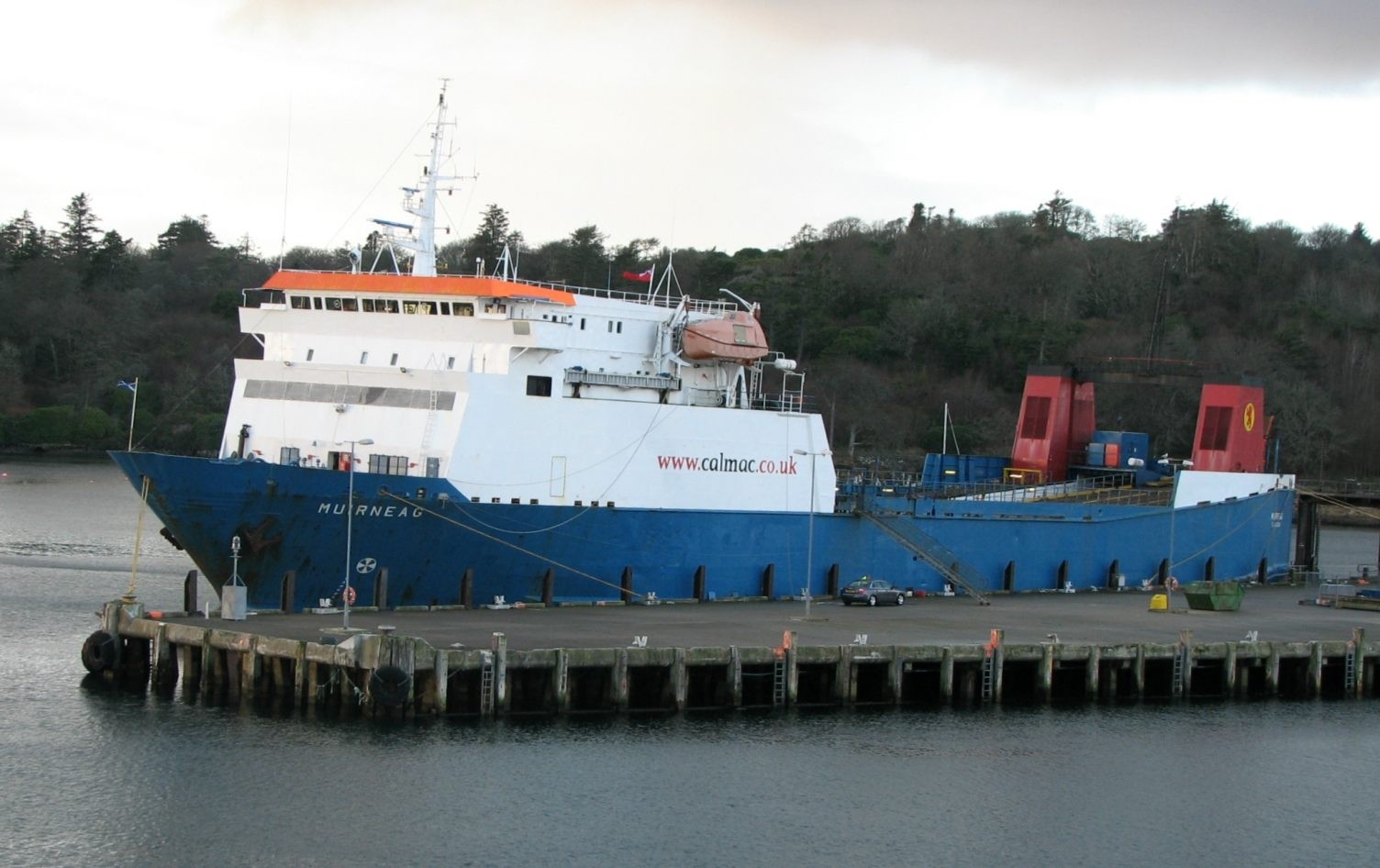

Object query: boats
[680,311,770,366]
[103,86,1303,608]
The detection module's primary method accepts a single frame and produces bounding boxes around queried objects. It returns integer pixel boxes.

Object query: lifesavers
[343,586,356,604]
[368,665,409,706]
[1165,576,1178,591]
[80,631,115,672]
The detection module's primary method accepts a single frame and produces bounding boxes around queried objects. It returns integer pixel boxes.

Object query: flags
[622,263,653,282]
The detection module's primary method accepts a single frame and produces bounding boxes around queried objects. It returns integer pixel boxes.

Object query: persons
[859,574,870,586]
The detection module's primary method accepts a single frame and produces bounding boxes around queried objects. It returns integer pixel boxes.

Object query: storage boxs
[1087,431,1151,468]
